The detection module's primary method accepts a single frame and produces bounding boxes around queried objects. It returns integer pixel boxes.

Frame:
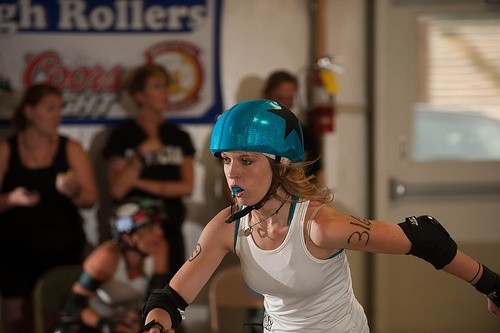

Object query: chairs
[208,265,264,333]
[33,265,81,333]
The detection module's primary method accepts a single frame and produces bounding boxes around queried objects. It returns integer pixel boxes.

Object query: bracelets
[158,180,165,195]
[68,182,83,200]
[132,145,148,168]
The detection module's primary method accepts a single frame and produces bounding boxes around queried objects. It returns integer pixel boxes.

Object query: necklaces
[20,127,53,168]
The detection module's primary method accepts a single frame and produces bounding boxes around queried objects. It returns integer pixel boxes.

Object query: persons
[50,192,177,333]
[138,97,500,333]
[102,61,198,287]
[260,70,323,188]
[0,82,100,333]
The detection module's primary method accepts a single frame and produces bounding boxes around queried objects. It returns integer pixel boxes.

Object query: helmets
[209,99,304,164]
[109,199,165,235]
